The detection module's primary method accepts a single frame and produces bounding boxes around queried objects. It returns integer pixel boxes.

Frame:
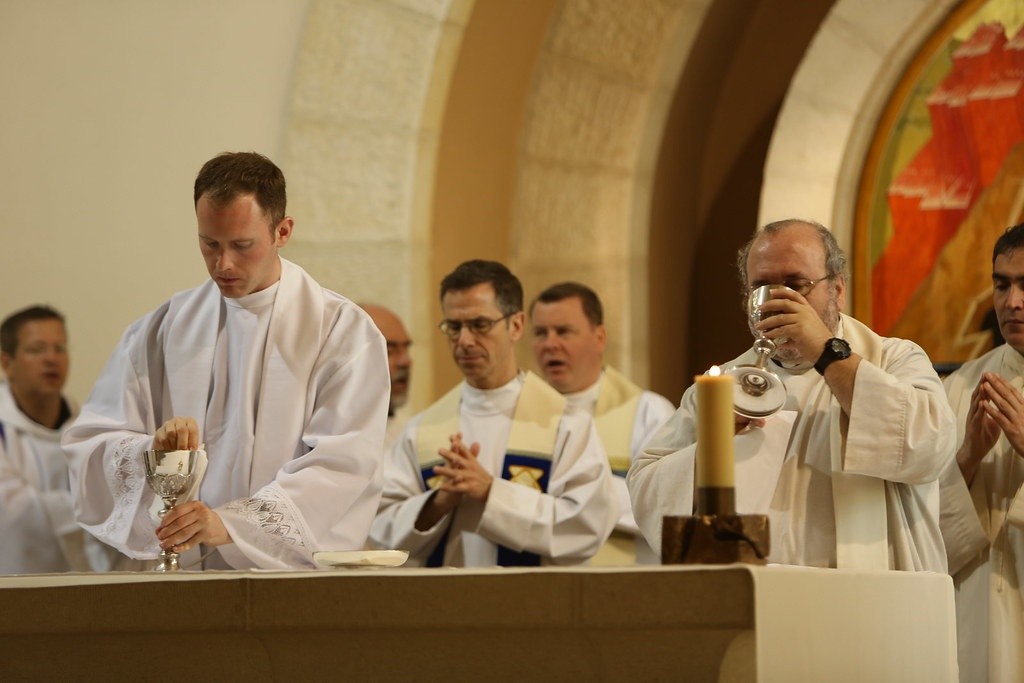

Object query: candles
[693,374,734,485]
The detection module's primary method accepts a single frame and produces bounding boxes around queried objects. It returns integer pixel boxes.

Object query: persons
[366,259,619,567]
[0,304,84,575]
[64,154,391,574]
[939,223,1024,683]
[624,219,961,683]
[527,282,675,568]
[354,300,412,421]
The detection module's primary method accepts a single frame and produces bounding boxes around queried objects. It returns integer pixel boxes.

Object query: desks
[0,568,959,683]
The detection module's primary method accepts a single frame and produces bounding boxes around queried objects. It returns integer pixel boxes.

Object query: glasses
[748,275,835,298]
[436,314,519,335]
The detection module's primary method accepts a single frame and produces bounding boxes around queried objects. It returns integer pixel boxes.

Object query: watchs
[813,337,852,376]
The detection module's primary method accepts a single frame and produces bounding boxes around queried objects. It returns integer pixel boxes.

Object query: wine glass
[722,285,795,419]
[144,449,199,574]
